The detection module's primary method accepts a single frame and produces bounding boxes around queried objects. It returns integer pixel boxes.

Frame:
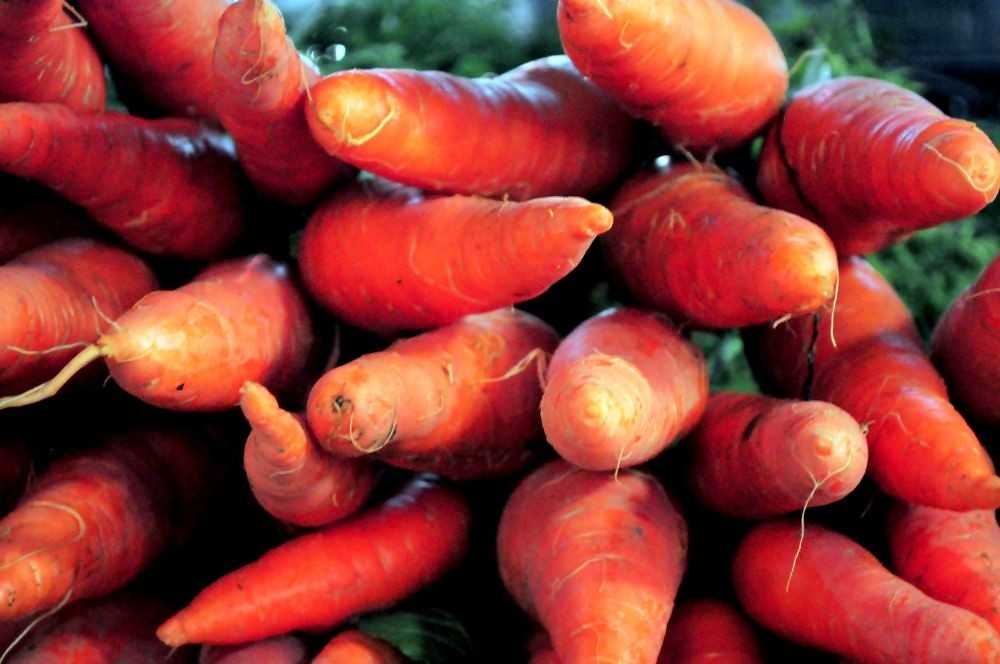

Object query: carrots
[0,0,1000,664]
